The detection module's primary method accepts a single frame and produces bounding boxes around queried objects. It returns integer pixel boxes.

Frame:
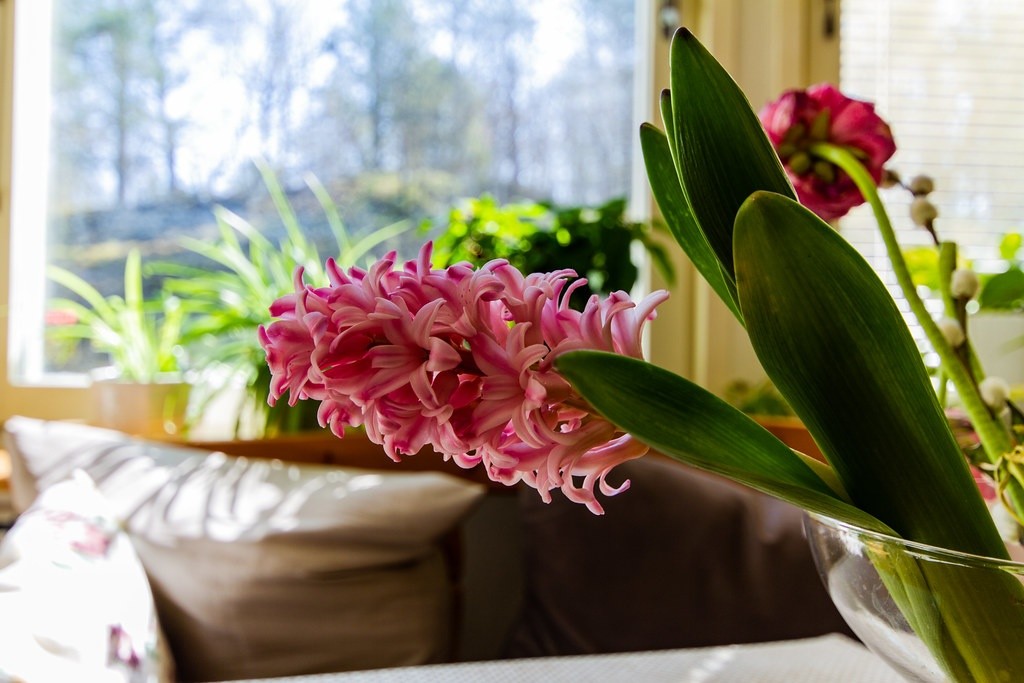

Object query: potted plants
[23,253,193,438]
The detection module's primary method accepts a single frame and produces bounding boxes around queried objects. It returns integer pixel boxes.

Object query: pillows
[0,469,173,683]
[5,413,481,678]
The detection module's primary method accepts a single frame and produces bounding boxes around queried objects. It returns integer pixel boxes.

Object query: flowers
[255,25,1024,683]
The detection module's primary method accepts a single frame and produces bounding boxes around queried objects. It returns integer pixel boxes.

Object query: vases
[801,510,1024,683]
